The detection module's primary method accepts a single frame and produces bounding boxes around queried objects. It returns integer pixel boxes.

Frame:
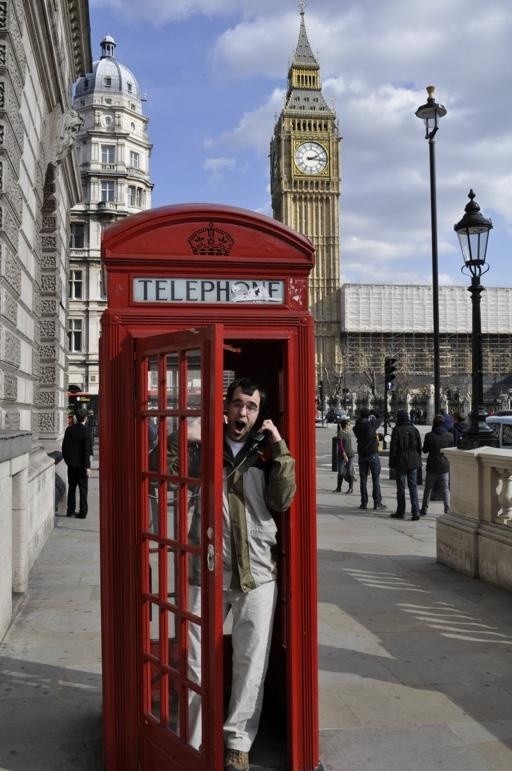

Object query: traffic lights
[386,358,396,382]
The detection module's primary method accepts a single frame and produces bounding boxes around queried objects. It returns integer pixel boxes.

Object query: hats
[360,408,371,417]
[397,410,409,422]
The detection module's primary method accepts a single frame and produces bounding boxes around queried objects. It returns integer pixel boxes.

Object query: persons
[62,409,91,518]
[333,408,472,520]
[148,378,297,771]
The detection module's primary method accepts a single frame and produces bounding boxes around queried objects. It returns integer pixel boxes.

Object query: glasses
[231,399,259,414]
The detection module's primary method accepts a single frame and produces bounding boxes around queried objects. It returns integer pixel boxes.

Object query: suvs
[325,409,350,424]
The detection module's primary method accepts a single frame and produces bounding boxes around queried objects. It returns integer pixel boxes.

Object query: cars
[315,412,326,423]
[485,407,512,449]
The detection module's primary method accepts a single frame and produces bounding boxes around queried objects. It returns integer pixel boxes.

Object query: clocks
[296,143,327,174]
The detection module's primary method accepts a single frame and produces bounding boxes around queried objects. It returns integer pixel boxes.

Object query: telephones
[246,412,270,455]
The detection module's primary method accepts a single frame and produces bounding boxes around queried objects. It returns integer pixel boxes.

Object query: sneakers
[390,512,405,520]
[419,506,428,515]
[346,488,354,494]
[360,502,368,510]
[410,512,420,521]
[374,500,388,511]
[75,513,86,519]
[333,487,341,493]
[444,504,450,514]
[67,510,78,517]
[224,743,250,770]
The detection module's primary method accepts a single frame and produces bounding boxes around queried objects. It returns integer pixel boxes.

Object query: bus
[69,387,231,438]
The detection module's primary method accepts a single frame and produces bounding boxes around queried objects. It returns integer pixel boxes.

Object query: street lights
[413,83,447,419]
[454,190,498,451]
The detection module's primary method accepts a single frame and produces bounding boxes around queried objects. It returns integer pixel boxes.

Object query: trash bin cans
[332,436,341,471]
